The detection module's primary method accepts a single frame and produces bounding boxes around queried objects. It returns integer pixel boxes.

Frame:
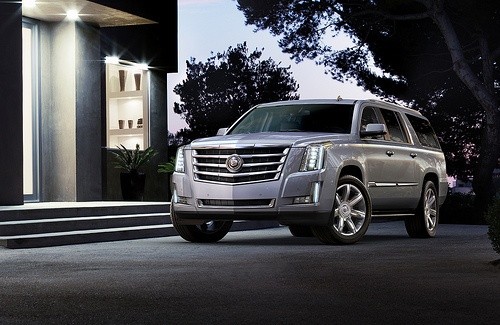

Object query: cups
[134,74,141,90]
[118,70,126,91]
[128,120,133,129]
[119,120,125,129]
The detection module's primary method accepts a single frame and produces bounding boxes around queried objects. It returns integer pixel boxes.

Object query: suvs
[169,96,449,245]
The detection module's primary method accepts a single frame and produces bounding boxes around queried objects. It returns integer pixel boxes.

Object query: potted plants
[109,145,175,201]
